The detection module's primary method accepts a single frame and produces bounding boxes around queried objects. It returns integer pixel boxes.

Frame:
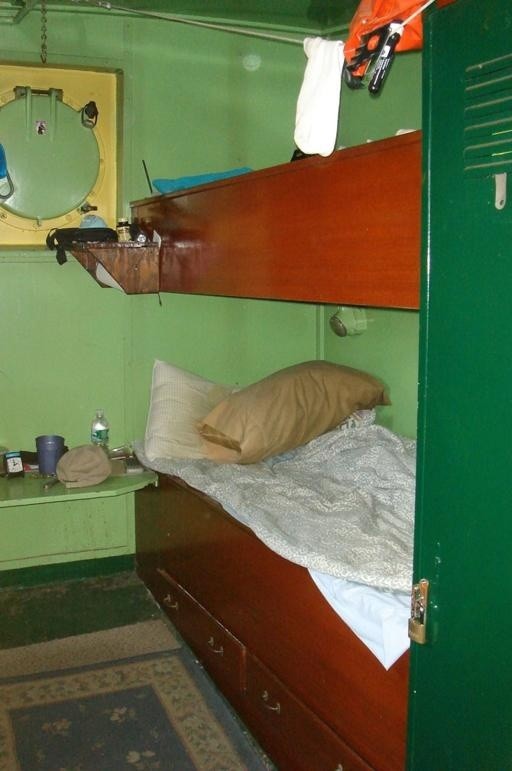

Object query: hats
[55,445,124,488]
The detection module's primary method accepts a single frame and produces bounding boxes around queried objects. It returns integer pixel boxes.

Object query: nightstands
[0,462,159,571]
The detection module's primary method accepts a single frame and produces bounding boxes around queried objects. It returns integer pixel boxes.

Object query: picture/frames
[0,58,124,251]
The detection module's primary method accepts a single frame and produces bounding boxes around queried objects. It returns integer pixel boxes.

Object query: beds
[129,127,421,312]
[135,409,417,771]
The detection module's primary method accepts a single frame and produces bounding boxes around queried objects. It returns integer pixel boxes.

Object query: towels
[294,36,345,158]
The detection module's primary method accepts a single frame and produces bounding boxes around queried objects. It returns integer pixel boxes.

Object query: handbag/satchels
[46,226,117,265]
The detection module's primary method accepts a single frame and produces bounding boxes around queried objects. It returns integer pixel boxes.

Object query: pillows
[197,360,393,464]
[144,358,238,462]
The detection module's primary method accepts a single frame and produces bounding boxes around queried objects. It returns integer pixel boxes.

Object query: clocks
[4,451,25,479]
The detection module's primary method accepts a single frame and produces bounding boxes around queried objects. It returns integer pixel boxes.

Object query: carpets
[0,570,266,771]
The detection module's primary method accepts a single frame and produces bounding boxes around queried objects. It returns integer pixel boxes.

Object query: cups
[35,436,63,478]
[117,218,131,240]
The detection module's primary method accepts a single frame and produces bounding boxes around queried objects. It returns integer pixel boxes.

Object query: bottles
[91,410,110,448]
